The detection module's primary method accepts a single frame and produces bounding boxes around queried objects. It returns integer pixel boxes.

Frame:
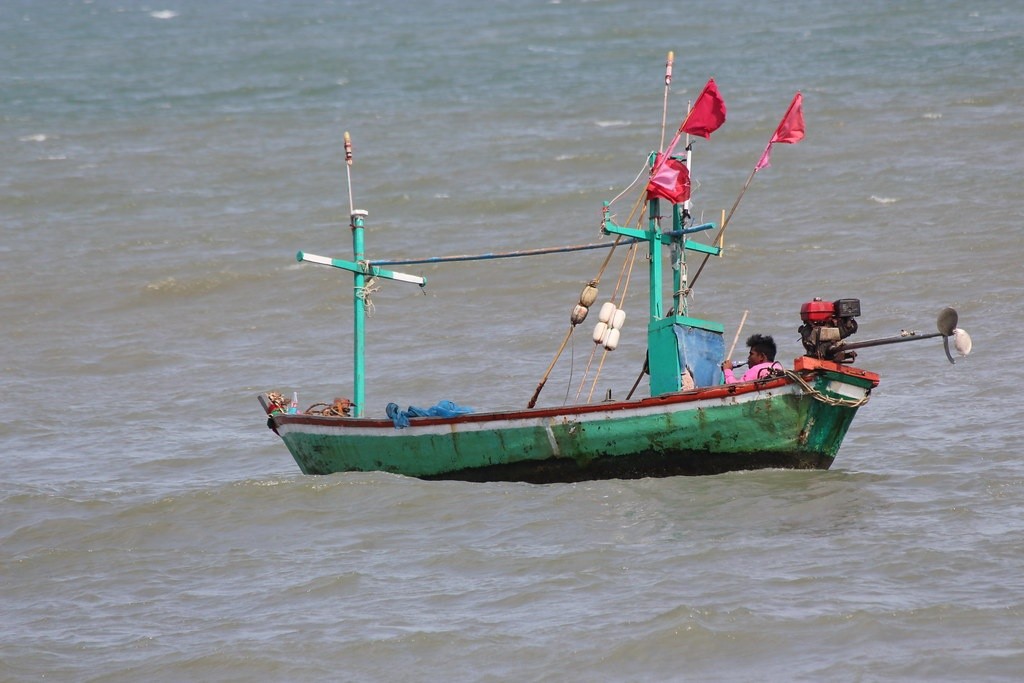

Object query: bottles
[721,361,749,371]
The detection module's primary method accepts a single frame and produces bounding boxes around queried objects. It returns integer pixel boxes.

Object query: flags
[646,81,805,206]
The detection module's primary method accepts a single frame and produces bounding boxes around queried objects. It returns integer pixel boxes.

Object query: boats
[254,41,973,477]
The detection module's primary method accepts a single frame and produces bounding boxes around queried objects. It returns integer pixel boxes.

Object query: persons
[721,334,783,386]
